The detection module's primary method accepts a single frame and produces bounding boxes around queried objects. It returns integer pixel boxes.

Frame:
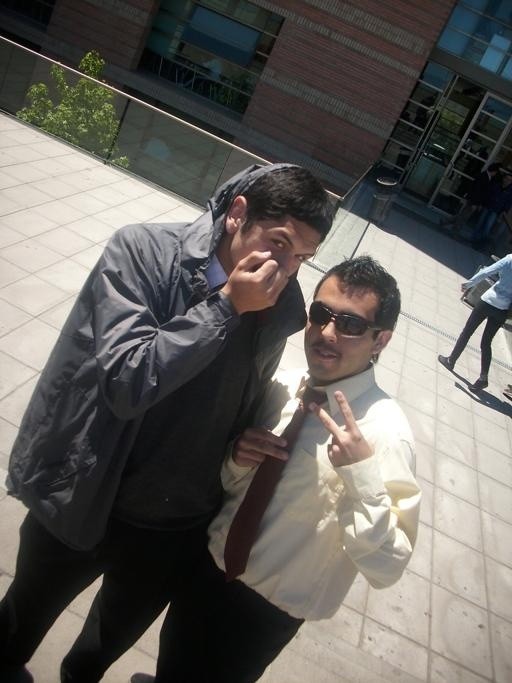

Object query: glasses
[308,299,383,337]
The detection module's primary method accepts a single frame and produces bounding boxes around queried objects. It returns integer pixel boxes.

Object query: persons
[130,254,423,683]
[438,250,512,393]
[0,162,335,683]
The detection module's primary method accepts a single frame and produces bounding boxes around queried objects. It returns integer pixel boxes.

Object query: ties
[222,383,329,584]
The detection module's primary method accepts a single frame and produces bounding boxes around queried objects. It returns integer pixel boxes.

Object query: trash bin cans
[368,176,400,224]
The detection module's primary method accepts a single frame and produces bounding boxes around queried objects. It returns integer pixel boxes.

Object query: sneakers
[469,379,488,393]
[438,354,455,372]
[130,673,155,683]
